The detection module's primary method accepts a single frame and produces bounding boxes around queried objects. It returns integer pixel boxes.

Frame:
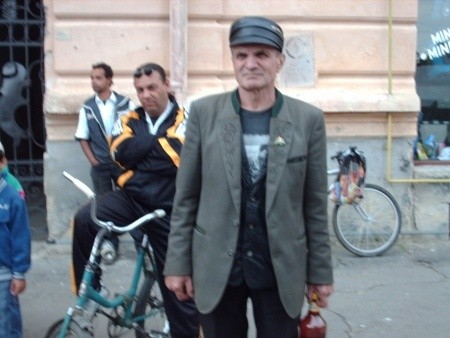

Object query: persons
[163,17,333,338]
[0,63,198,338]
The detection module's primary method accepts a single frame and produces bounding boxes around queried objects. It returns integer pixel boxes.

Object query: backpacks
[327,148,368,207]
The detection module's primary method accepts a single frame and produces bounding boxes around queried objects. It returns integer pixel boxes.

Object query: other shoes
[77,285,109,331]
[100,239,118,265]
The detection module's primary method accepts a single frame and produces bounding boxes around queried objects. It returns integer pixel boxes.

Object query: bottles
[300,293,327,338]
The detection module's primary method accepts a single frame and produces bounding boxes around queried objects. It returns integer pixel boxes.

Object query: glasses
[133,67,153,78]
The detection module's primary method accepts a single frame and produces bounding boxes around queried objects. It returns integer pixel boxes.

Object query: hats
[229,15,284,53]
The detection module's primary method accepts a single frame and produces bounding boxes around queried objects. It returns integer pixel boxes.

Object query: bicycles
[45,169,173,335]
[326,144,404,257]
